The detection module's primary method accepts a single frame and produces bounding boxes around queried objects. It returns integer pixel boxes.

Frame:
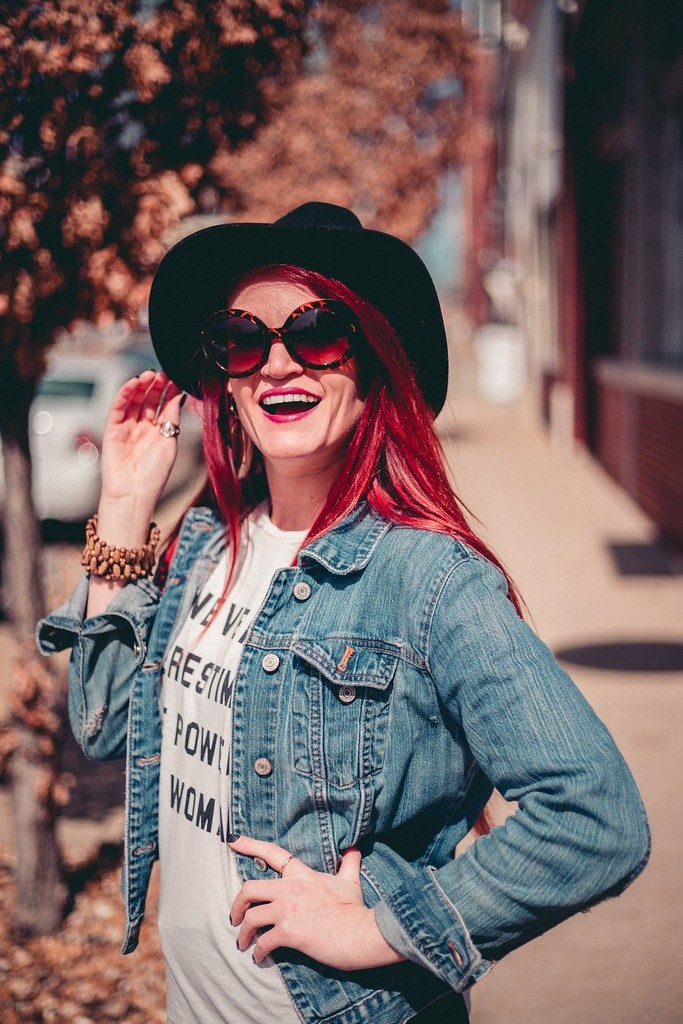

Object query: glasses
[198,299,363,378]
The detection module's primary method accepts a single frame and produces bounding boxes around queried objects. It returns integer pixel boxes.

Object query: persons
[36,201,653,1024]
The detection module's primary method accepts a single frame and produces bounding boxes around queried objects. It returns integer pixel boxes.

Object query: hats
[147,201,449,421]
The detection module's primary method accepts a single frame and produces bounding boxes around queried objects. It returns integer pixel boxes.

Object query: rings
[159,422,182,438]
[279,856,294,874]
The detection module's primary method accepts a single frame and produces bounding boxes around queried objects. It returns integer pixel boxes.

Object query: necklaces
[310,497,325,528]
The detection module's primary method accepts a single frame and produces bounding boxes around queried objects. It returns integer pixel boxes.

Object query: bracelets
[80,514,160,581]
